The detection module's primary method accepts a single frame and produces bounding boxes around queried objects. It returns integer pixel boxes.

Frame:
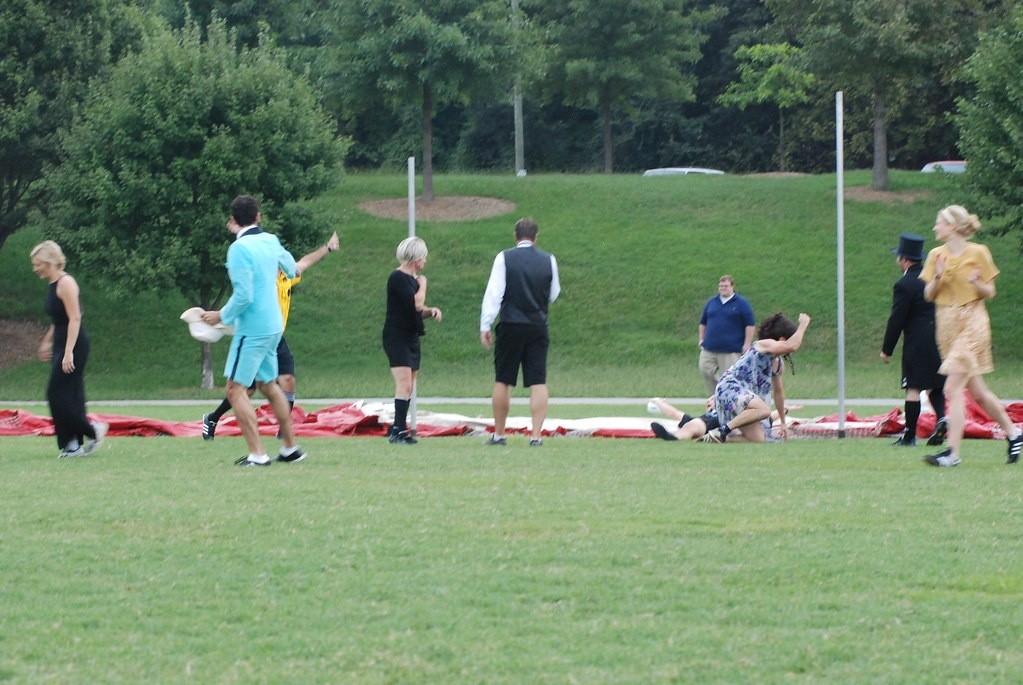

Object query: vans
[920,160,969,174]
[641,167,726,176]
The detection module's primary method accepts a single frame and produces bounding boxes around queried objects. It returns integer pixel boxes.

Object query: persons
[202,230,342,442]
[916,206,1022,467]
[199,196,306,468]
[30,239,104,458]
[382,236,441,445]
[479,216,561,447]
[879,234,948,446]
[651,312,812,444]
[697,274,757,398]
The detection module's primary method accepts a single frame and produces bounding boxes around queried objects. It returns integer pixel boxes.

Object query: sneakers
[1004,430,1023,463]
[891,436,917,448]
[928,417,948,447]
[275,429,283,439]
[56,445,85,459]
[927,450,963,466]
[201,412,218,440]
[388,426,418,444]
[237,457,271,466]
[82,424,104,456]
[272,448,307,463]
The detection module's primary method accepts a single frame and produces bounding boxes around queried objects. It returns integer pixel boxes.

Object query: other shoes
[708,428,726,442]
[697,433,714,442]
[651,422,678,440]
[484,434,506,445]
[529,439,542,446]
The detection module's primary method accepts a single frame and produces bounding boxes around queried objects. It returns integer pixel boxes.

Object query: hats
[891,233,926,260]
[180,307,233,344]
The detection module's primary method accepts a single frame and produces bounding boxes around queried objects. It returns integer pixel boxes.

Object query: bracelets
[935,273,941,280]
[325,243,332,252]
